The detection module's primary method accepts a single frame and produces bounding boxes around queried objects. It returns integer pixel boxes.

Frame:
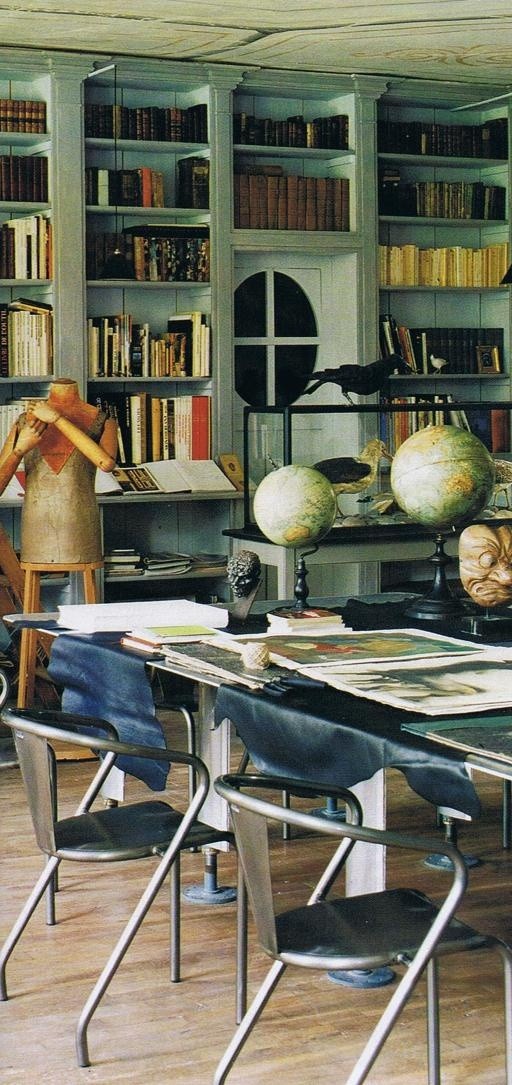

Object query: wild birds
[492,457,512,510]
[264,439,395,518]
[298,352,415,408]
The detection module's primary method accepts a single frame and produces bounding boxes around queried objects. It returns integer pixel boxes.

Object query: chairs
[213,774,511,1085]
[0,708,248,1068]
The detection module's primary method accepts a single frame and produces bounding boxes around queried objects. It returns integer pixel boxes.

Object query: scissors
[243,674,298,697]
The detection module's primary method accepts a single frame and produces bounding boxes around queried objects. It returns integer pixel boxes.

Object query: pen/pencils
[280,679,325,689]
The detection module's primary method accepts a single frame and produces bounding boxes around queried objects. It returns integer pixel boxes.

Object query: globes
[254,465,336,627]
[390,426,498,621]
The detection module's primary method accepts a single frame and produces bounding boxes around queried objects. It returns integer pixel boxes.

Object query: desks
[0,591,511,989]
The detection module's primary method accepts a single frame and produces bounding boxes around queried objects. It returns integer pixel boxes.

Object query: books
[378,164,505,220]
[379,314,504,374]
[232,112,349,150]
[0,97,54,281]
[88,391,236,495]
[265,608,354,636]
[376,392,511,452]
[233,163,350,231]
[104,547,228,577]
[0,296,52,464]
[85,101,210,282]
[86,310,210,377]
[379,240,510,287]
[119,624,235,654]
[379,118,509,159]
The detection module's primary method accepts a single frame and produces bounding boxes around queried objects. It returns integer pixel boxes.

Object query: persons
[0,377,117,565]
